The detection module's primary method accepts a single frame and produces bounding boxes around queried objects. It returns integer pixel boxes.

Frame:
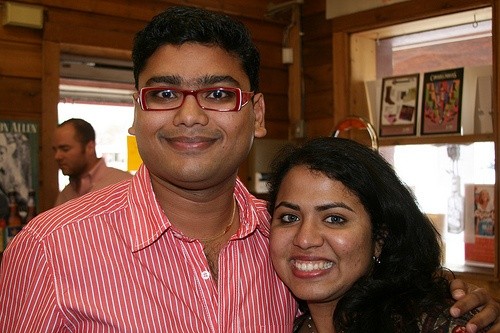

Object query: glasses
[135,86,255,112]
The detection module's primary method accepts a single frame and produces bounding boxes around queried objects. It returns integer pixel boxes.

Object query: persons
[0,5,500,333]
[51,118,133,207]
[266,137,493,333]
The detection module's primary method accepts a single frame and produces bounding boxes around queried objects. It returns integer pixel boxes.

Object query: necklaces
[200,195,237,242]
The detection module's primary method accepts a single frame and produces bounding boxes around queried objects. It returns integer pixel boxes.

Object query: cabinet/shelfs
[329,0,500,297]
